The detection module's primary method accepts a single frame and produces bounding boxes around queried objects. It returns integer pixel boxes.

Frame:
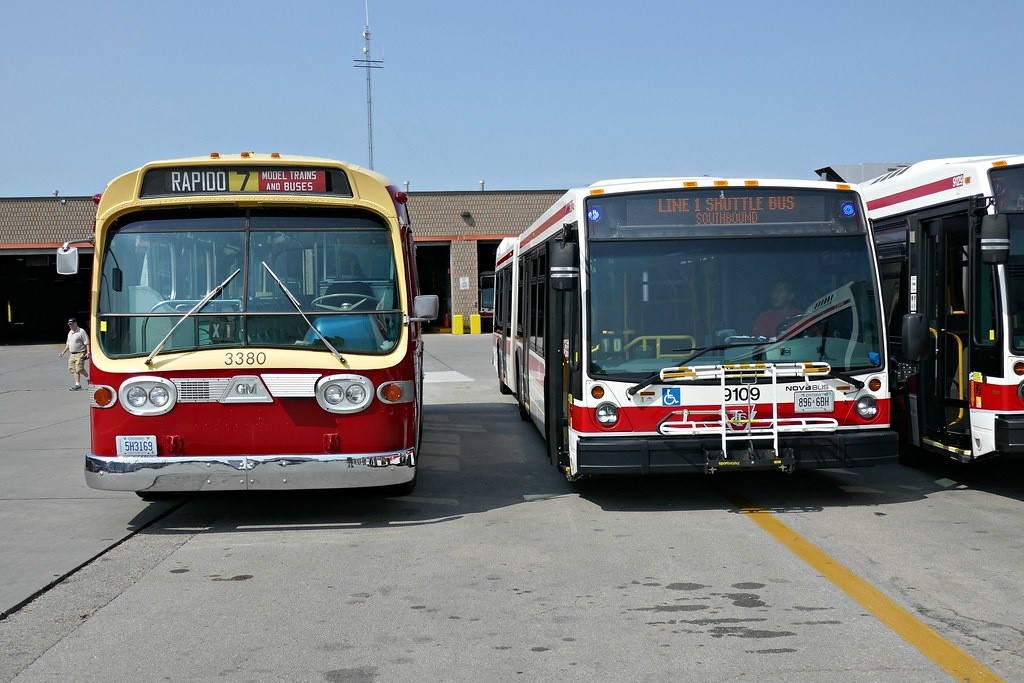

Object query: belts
[70,352,80,354]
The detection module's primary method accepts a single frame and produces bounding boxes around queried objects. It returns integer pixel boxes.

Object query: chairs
[318,282,377,311]
[764,279,825,334]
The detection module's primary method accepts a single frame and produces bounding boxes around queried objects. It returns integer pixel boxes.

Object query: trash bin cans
[470,314,481,334]
[452,314,463,334]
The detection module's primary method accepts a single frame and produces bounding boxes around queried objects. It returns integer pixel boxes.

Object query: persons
[750,279,822,342]
[59,318,90,391]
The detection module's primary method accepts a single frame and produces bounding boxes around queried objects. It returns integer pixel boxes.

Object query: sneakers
[86,379,90,386]
[69,384,82,391]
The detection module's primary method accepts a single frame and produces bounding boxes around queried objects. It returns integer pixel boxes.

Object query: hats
[67,318,76,325]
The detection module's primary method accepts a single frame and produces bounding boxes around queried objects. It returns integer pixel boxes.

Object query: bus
[490,177,897,492]
[57,152,440,497]
[853,155,1024,482]
